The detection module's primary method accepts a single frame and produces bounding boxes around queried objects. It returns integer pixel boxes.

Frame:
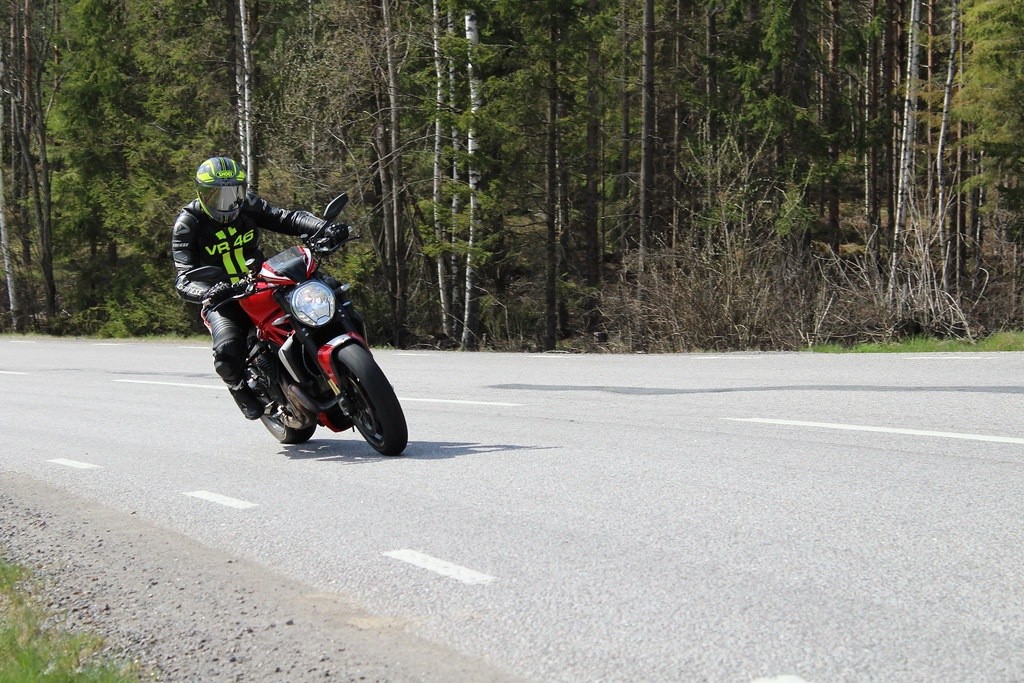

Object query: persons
[171,157,349,419]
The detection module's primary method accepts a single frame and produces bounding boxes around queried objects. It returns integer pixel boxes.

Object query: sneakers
[228,379,264,420]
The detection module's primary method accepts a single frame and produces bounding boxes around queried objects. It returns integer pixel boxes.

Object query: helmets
[195,157,247,224]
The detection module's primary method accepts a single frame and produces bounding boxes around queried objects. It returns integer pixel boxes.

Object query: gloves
[324,223,349,245]
[204,282,230,304]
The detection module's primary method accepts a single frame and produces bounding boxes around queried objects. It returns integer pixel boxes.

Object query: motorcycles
[186,192,408,456]
